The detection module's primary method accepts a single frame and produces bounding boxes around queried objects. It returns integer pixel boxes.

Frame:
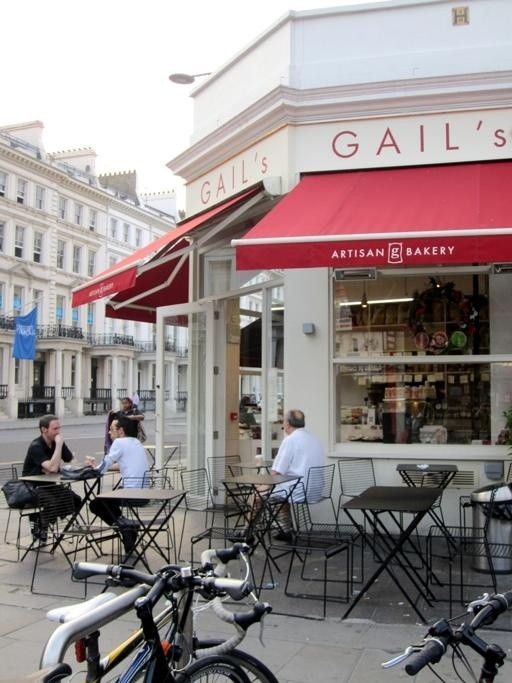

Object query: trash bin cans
[470,482,512,575]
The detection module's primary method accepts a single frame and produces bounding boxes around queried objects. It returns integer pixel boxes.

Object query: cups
[385,380,437,400]
[255,454,263,467]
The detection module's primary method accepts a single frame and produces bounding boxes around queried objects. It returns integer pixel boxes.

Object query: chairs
[0,455,512,625]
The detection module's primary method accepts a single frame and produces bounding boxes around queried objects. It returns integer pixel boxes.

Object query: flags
[12,306,39,361]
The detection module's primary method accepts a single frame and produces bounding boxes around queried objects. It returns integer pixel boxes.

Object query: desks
[143,443,187,489]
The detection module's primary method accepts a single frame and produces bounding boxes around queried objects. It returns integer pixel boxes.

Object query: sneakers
[38,520,48,541]
[123,526,139,553]
[228,532,255,544]
[273,530,293,542]
[29,513,41,537]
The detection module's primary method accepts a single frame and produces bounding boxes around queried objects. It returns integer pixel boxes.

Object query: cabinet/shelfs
[336,302,490,411]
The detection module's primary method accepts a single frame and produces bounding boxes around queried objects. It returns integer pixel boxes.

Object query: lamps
[362,282,368,307]
[339,278,414,306]
[168,72,211,84]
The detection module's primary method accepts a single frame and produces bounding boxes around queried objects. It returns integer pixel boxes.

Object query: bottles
[362,396,381,425]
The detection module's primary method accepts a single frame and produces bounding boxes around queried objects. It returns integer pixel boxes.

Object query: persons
[85,416,153,556]
[23,414,74,543]
[110,396,144,439]
[131,391,140,410]
[239,395,257,430]
[226,409,324,545]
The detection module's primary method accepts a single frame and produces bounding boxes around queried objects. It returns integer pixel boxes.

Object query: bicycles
[381,590,511,682]
[10,542,280,682]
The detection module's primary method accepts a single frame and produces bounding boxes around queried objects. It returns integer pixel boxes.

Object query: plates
[415,331,467,349]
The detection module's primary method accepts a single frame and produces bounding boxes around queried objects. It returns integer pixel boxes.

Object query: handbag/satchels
[4,480,40,506]
[63,465,96,478]
[137,421,146,442]
